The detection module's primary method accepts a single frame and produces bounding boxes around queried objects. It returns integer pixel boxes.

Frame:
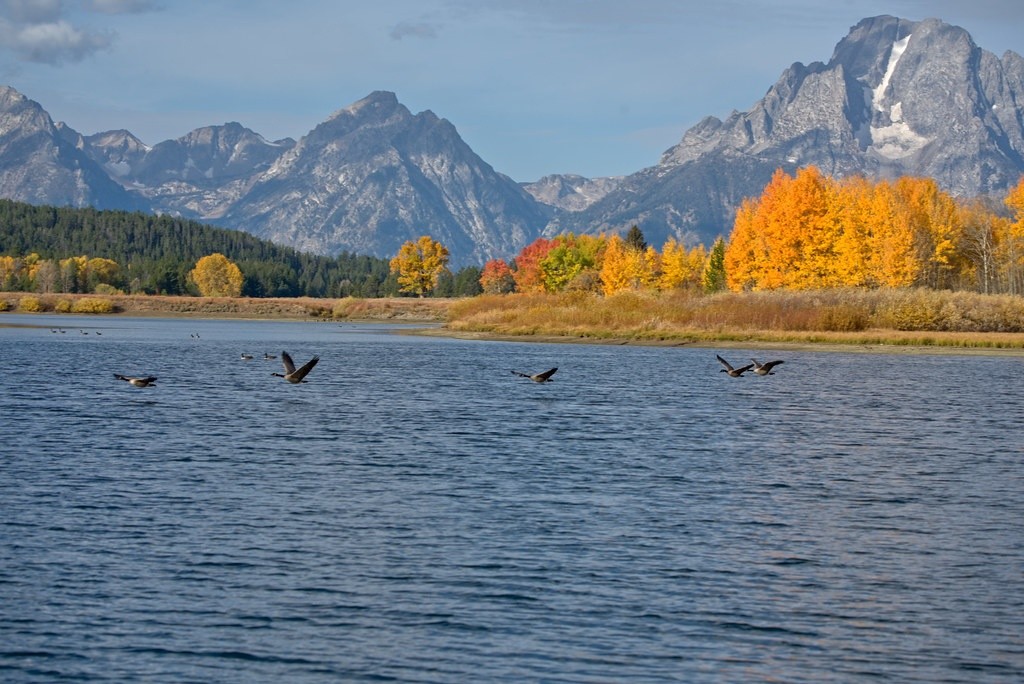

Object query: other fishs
[48,328,57,333]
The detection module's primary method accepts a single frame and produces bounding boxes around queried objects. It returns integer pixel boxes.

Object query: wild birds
[96,332,102,335]
[113,373,158,387]
[59,328,66,333]
[191,334,194,338]
[716,355,755,377]
[747,358,786,375]
[511,367,559,383]
[80,330,89,335]
[271,350,321,384]
[265,353,278,360]
[196,333,199,337]
[241,353,253,360]
